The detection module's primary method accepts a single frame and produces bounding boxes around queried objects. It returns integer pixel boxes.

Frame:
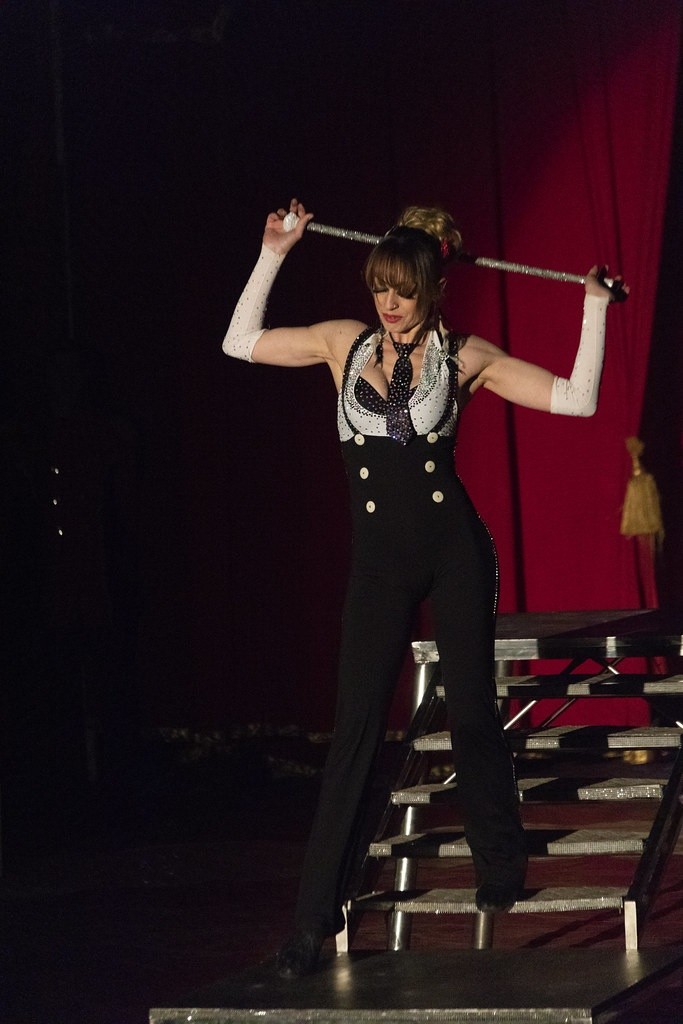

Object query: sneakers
[274,911,346,983]
[475,835,528,914]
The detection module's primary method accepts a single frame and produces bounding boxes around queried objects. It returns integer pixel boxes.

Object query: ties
[386,338,418,447]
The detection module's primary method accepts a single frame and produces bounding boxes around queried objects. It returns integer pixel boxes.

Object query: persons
[221,198,630,973]
